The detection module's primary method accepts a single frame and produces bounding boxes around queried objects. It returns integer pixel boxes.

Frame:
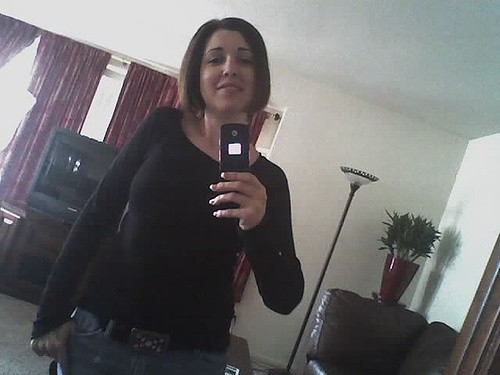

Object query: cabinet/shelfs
[0,199,73,307]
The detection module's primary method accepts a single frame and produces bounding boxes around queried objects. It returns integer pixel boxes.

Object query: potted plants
[376,208,443,305]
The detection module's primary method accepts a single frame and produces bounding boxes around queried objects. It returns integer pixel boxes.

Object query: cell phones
[220,123,250,181]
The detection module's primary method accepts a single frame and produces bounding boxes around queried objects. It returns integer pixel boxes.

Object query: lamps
[265,166,380,375]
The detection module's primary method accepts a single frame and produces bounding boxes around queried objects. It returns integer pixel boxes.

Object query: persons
[28,16,309,374]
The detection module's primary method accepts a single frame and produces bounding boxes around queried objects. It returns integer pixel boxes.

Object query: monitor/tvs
[25,125,120,226]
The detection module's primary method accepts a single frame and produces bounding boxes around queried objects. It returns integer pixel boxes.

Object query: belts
[72,305,230,356]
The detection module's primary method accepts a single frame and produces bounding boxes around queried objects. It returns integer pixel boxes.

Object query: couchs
[304,288,459,375]
